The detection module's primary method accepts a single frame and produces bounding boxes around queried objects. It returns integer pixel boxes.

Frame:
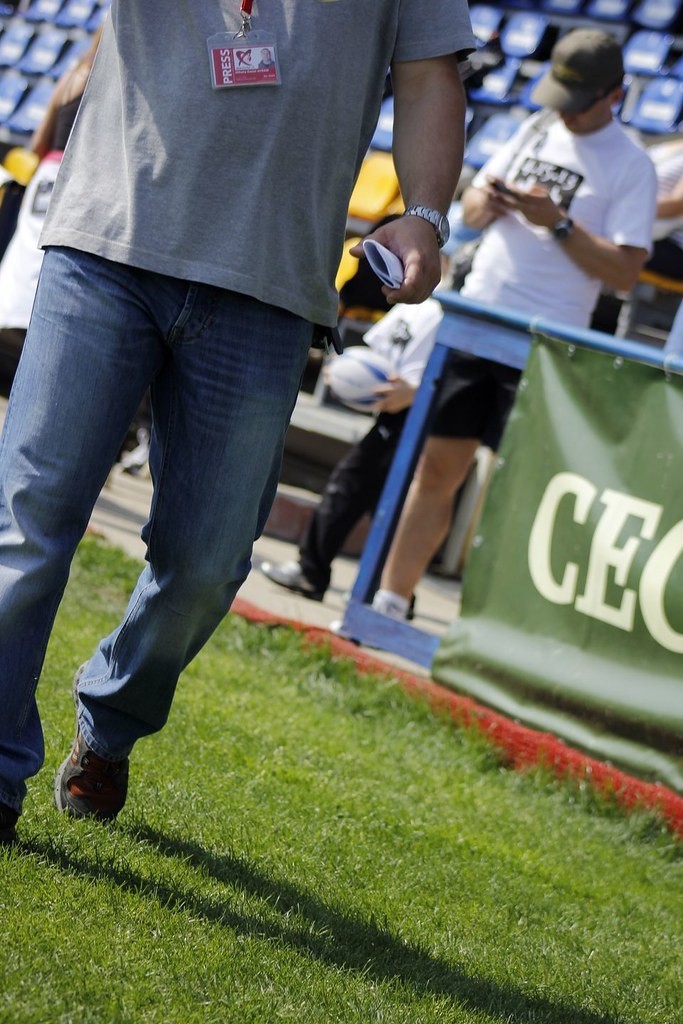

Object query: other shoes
[260,560,324,602]
[329,591,407,651]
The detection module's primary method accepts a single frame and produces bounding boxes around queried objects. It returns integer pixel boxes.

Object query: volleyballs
[328,344,396,415]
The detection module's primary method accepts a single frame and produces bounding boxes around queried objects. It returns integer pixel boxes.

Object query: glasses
[576,83,613,116]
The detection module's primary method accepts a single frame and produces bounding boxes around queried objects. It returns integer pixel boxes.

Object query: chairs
[0,0,683,577]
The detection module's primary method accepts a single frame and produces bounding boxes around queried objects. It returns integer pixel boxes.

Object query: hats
[530,29,624,114]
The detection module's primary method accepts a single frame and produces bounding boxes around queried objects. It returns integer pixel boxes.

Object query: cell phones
[484,175,509,194]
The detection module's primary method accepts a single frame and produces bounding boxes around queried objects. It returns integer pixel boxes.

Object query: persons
[257,173,446,621]
[258,48,274,70]
[0,0,475,845]
[374,29,657,626]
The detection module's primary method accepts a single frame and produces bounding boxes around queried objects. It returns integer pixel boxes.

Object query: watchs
[551,219,573,241]
[404,205,450,248]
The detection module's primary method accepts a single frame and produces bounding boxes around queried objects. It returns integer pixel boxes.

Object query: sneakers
[0,802,19,845]
[55,660,130,826]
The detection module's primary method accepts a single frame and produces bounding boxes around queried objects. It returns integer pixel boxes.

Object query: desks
[337,292,683,667]
